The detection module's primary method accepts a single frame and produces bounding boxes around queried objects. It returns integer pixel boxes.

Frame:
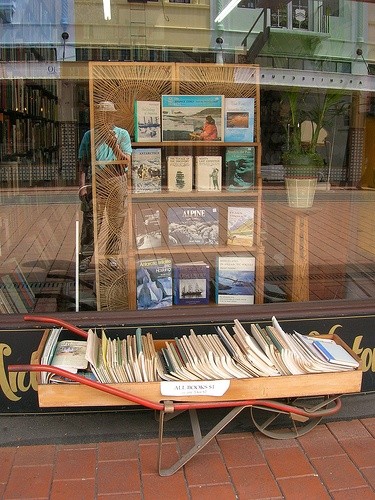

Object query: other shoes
[107,260,119,270]
[80,262,89,273]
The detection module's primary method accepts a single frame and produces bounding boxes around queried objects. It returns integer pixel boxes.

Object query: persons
[196,116,217,141]
[78,101,132,273]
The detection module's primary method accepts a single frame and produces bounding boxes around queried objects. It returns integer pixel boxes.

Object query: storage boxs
[35,333,362,408]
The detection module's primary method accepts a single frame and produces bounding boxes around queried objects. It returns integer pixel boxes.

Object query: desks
[269,201,316,304]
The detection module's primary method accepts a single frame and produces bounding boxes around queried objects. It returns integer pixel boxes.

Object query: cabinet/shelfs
[125,141,263,310]
[0,0,58,159]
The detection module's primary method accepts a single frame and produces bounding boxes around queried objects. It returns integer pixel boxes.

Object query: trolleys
[34,273,294,310]
[6,316,361,478]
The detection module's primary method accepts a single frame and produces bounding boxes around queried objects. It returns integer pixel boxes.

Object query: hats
[97,101,117,112]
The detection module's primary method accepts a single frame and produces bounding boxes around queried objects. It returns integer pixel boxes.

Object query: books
[41,316,359,384]
[136,258,172,310]
[135,206,162,249]
[224,98,254,142]
[215,254,255,305]
[133,101,161,142]
[174,260,210,305]
[166,156,192,192]
[195,156,222,192]
[162,95,225,142]
[226,146,255,192]
[132,148,162,194]
[227,206,255,247]
[0,256,37,313]
[167,207,220,247]
[0,0,77,202]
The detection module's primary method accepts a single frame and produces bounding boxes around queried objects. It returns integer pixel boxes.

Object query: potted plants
[253,33,348,206]
